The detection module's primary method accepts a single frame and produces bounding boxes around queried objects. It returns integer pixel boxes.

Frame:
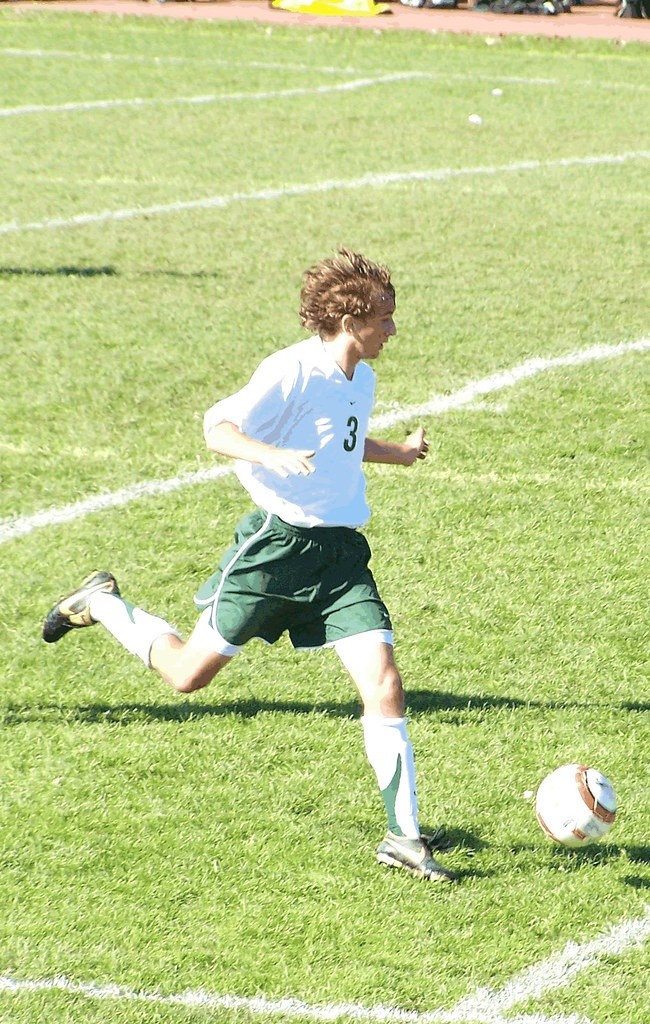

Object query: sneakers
[42,571,121,643]
[376,830,460,883]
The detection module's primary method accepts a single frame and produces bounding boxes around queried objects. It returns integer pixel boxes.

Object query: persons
[40,245,463,888]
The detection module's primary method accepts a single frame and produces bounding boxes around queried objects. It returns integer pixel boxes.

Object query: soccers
[534,764,619,849]
